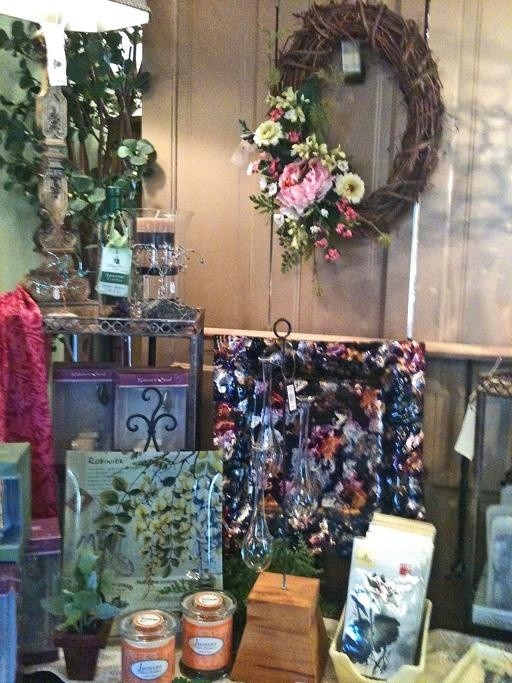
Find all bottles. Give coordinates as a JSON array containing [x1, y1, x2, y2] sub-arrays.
[[122, 587, 237, 683]]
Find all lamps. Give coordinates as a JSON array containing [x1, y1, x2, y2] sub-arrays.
[[0, 0, 153, 306]]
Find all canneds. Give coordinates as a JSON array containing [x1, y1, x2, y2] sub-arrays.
[[119, 610, 178, 683], [178, 589, 237, 681]]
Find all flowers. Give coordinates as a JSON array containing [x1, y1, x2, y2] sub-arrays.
[[231, 26, 397, 275]]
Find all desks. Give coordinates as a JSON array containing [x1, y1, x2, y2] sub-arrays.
[[9, 612, 511, 681], [36, 300, 209, 453]]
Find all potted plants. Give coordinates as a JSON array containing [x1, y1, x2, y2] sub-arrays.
[[40, 549, 129, 682]]
